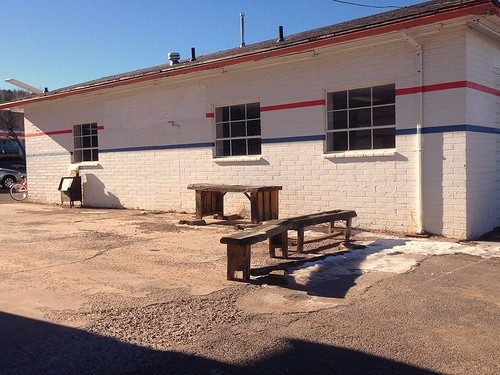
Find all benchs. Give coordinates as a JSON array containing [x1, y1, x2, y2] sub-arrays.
[[283, 209, 359, 253], [220, 219, 290, 283]]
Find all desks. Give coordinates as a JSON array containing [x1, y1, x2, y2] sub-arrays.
[[187, 182, 283, 224]]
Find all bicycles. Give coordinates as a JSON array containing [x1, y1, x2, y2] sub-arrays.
[[10, 176, 28, 201]]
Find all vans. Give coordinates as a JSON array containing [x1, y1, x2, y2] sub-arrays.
[[0, 137, 26, 190]]
